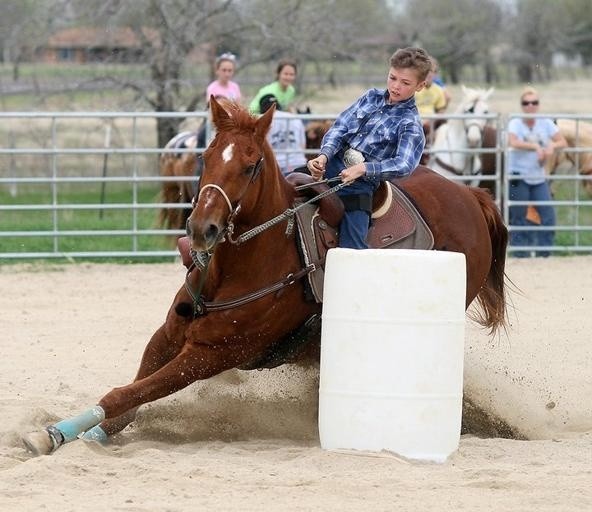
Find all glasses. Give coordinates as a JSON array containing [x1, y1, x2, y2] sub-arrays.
[[522, 100, 538, 106]]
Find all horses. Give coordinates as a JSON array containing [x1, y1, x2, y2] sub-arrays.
[[554, 119, 592, 199], [22, 92, 532, 458], [150, 83, 508, 250]]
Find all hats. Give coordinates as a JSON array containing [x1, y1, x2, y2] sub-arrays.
[[259, 94, 279, 112]]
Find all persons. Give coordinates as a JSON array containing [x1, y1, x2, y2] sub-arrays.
[[206, 50, 241, 117], [505, 86, 568, 257], [308, 46, 434, 249], [249, 60, 298, 112], [413, 56, 451, 114]]
